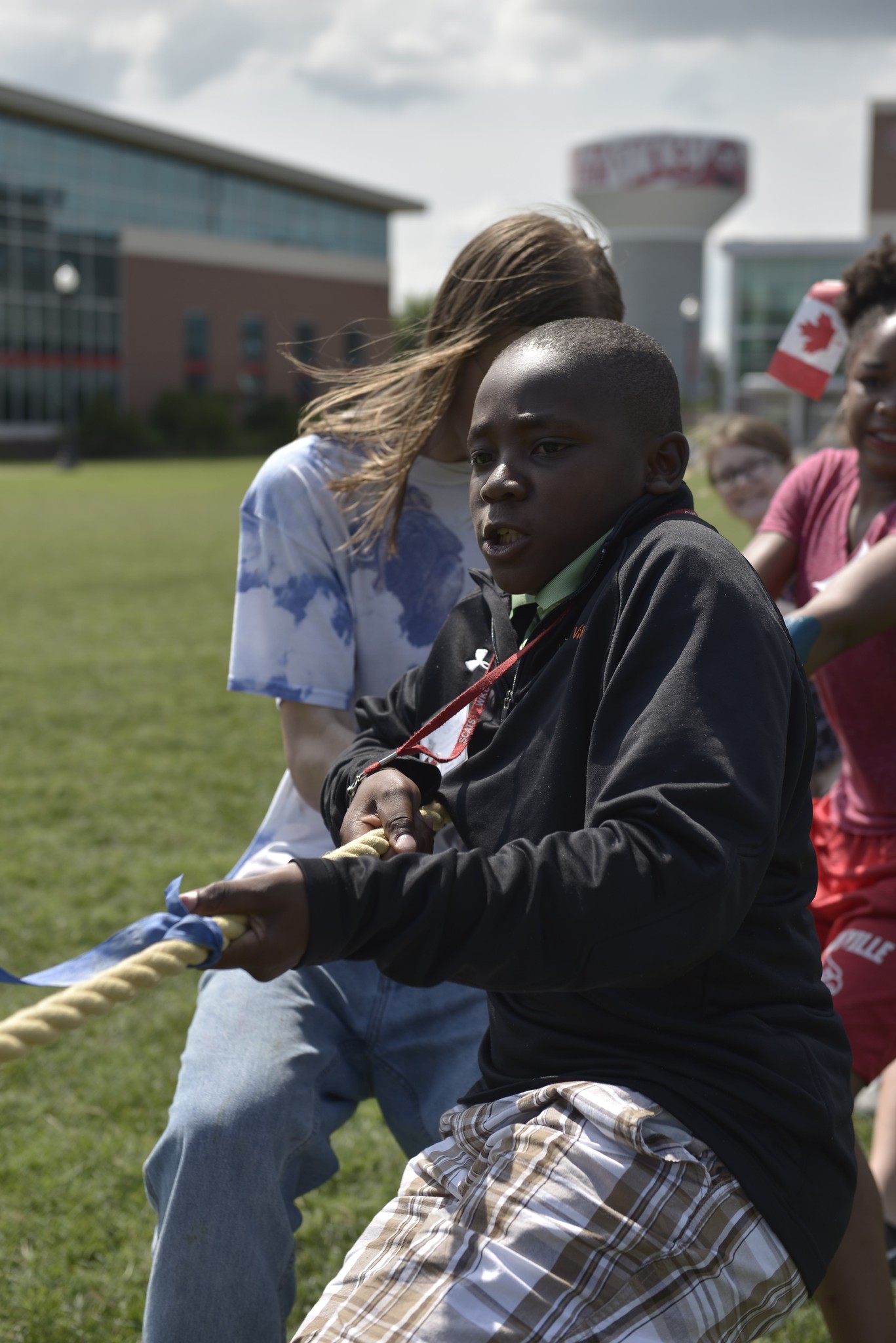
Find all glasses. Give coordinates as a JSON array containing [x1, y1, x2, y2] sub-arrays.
[[709, 455, 778, 492]]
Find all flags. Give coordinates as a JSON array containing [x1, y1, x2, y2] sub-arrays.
[[767, 278, 846, 401]]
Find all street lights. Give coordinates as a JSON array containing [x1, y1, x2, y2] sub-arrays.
[[52, 262, 81, 469], [680, 296, 700, 435]]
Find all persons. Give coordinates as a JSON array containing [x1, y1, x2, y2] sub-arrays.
[[739, 234, 896, 1234], [143, 213, 623, 1343], [178, 320, 853, 1343], [707, 417, 796, 533]]
